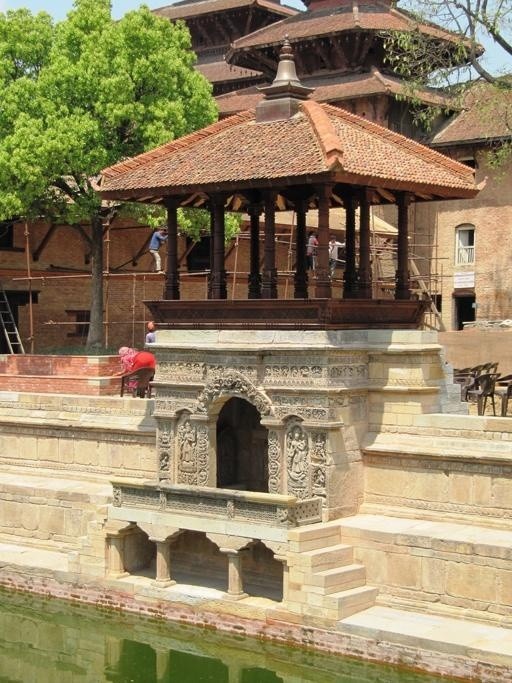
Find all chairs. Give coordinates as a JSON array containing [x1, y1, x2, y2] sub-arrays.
[[454, 362, 512, 416], [121, 367, 155, 399]]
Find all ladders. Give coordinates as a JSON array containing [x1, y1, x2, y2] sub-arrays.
[[0, 289, 25, 354]]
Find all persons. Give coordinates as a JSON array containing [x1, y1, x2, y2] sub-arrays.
[[306, 233, 319, 271], [115, 347, 140, 393], [148, 226, 168, 274], [328, 234, 346, 280], [144, 321, 156, 354]]
